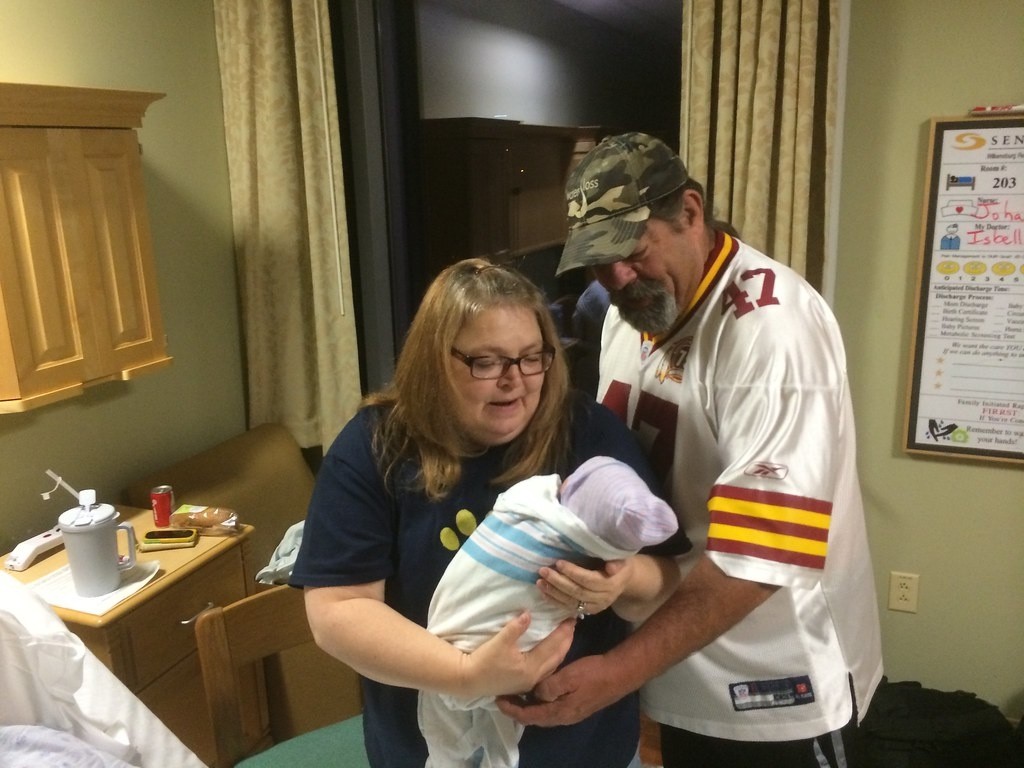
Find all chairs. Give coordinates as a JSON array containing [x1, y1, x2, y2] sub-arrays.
[[193, 584, 371, 768]]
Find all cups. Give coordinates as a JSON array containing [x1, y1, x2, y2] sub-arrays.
[[59, 503, 136, 596]]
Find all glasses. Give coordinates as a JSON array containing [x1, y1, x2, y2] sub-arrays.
[[450, 339, 555, 380]]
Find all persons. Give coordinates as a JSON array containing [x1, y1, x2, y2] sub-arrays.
[[417, 454, 678, 768], [553, 133, 882, 768], [291, 256, 692, 768]]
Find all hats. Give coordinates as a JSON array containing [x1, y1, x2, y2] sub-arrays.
[[553, 132, 689, 274], [559, 455, 679, 551]]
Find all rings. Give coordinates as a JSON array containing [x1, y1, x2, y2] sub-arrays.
[[575, 602, 589, 619]]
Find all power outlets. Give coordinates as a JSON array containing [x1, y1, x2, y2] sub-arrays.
[[886, 570, 920, 615]]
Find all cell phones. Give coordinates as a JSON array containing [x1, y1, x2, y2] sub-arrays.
[[142, 528, 197, 543]]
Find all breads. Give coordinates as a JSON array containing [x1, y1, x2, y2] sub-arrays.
[[169, 504, 241, 535]]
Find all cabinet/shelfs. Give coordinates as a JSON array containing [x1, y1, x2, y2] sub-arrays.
[[0, 81, 174, 414], [0, 505, 274, 768], [422, 116, 615, 267]]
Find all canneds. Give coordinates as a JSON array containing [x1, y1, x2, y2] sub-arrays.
[[150, 484, 174, 527]]
